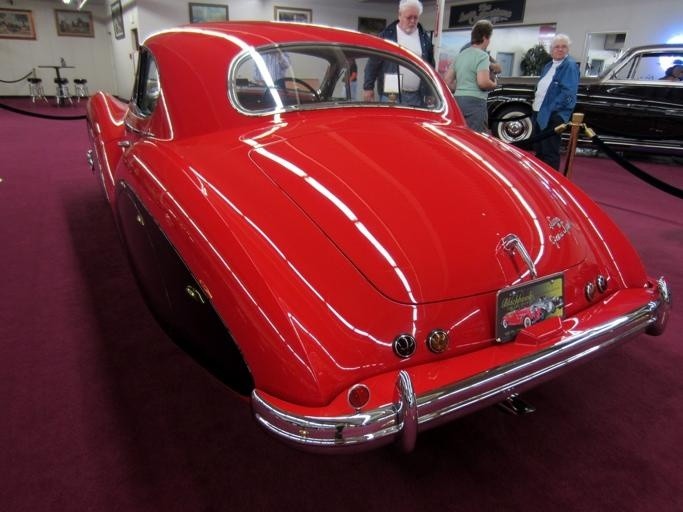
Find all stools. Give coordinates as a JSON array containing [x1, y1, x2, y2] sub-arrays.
[[26, 77, 89, 106]]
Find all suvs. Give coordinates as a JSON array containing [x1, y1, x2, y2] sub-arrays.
[[487, 43, 682, 158]]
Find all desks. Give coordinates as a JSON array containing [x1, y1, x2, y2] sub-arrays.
[[39, 64, 76, 107]]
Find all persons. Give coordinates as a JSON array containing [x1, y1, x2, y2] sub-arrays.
[[658, 65, 683, 82], [531, 33, 579, 172], [342, 60, 358, 101], [250, 52, 291, 88], [444, 20, 502, 134], [363, 0, 436, 108]]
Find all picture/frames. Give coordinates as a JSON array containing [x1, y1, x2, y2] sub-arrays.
[[110, 1, 125, 38], [1, 9, 36, 41], [189, 3, 228, 23], [274, 6, 312, 24], [54, 9, 95, 37]]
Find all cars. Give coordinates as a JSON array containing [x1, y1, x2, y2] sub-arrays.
[[83, 20, 670, 458]]
